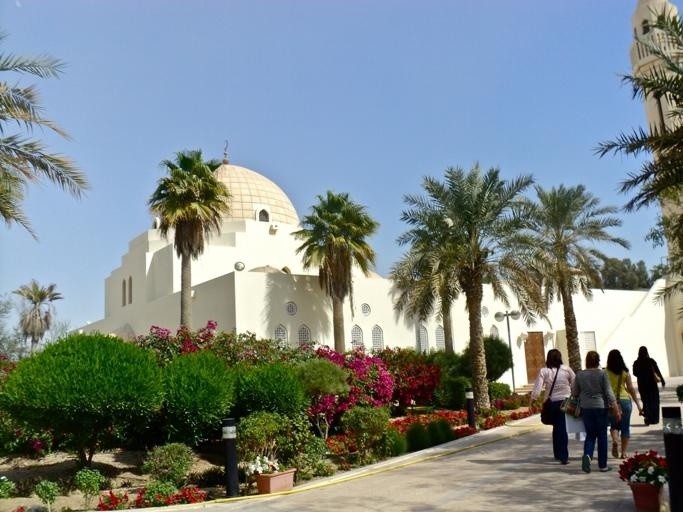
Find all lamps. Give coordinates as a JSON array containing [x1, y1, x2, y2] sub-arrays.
[[519, 332, 528, 343], [546, 331, 554, 338]]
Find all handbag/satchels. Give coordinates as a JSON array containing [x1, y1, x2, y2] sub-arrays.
[[565, 412, 586, 433], [559, 394, 582, 418], [541, 400, 554, 424], [654, 372, 661, 382], [608, 403, 622, 419]]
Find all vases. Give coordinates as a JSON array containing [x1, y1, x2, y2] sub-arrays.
[[629, 483, 661, 512], [256, 467, 297, 494]]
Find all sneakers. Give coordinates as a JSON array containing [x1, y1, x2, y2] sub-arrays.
[[612, 441, 628, 458], [555, 456, 570, 464], [582, 454, 592, 473], [600, 467, 612, 472]]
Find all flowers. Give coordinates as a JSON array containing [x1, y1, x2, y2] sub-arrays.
[[241, 456, 288, 476], [618, 449, 672, 486]]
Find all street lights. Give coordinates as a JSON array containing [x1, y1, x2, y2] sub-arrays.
[[495, 310, 520, 393]]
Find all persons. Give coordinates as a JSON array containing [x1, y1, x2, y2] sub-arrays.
[[632, 346, 665, 425], [573, 351, 622, 473], [530, 348, 576, 465], [603, 349, 642, 458]]
